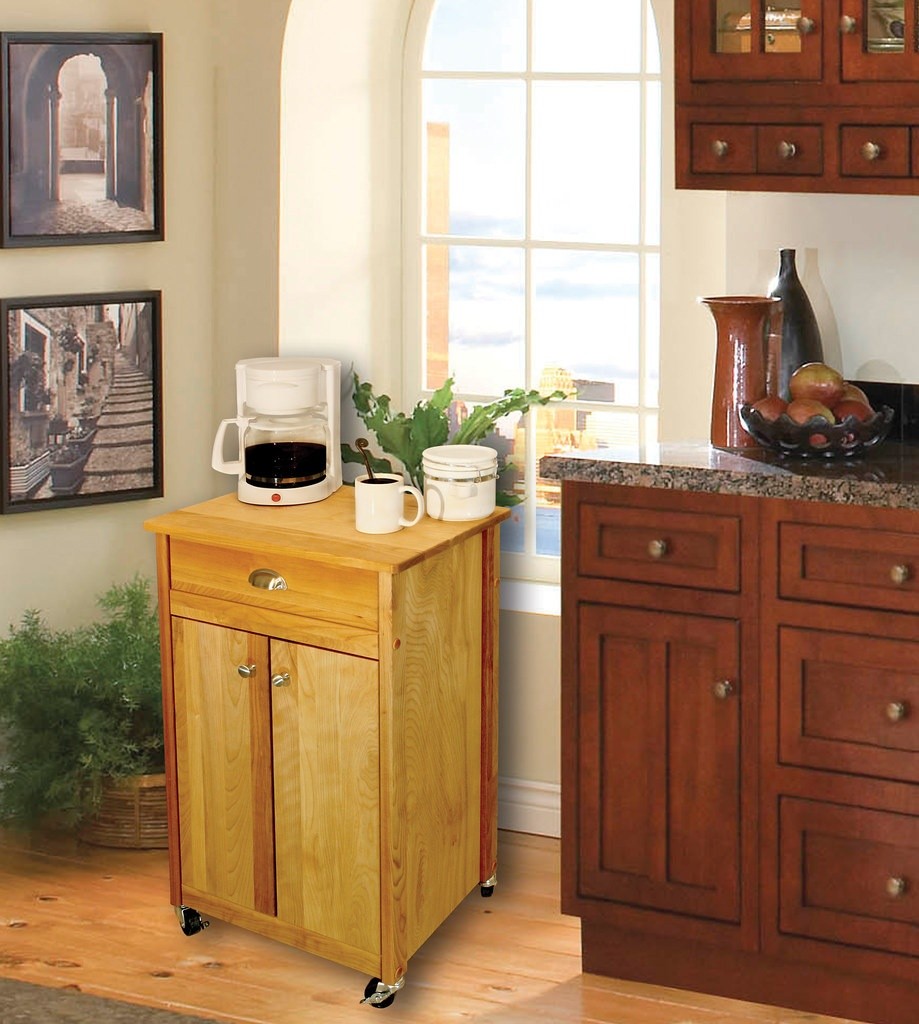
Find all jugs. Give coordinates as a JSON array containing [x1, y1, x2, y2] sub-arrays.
[[211, 409, 328, 486]]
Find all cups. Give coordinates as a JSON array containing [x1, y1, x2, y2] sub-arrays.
[[353, 473, 425, 534]]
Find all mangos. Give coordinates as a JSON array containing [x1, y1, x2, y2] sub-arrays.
[[790, 364, 849, 407], [833, 402, 874, 442], [751, 397, 787, 424], [843, 386, 869, 403], [787, 400, 835, 445]]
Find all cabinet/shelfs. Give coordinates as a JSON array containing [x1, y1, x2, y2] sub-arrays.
[[142, 482, 510, 1012], [561, 488, 918, 1024], [672, 0, 919, 196]]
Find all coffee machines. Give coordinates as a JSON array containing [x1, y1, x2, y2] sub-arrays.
[[236, 356, 344, 508]]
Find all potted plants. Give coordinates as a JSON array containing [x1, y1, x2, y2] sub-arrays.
[[0, 568, 168, 854]]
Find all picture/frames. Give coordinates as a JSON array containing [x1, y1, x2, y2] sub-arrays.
[[0, 31, 165, 249], [0, 288, 164, 514]]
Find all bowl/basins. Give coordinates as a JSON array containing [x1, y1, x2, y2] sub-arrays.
[[422, 444, 498, 522]]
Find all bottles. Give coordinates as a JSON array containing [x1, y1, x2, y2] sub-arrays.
[[765, 247, 822, 403]]
